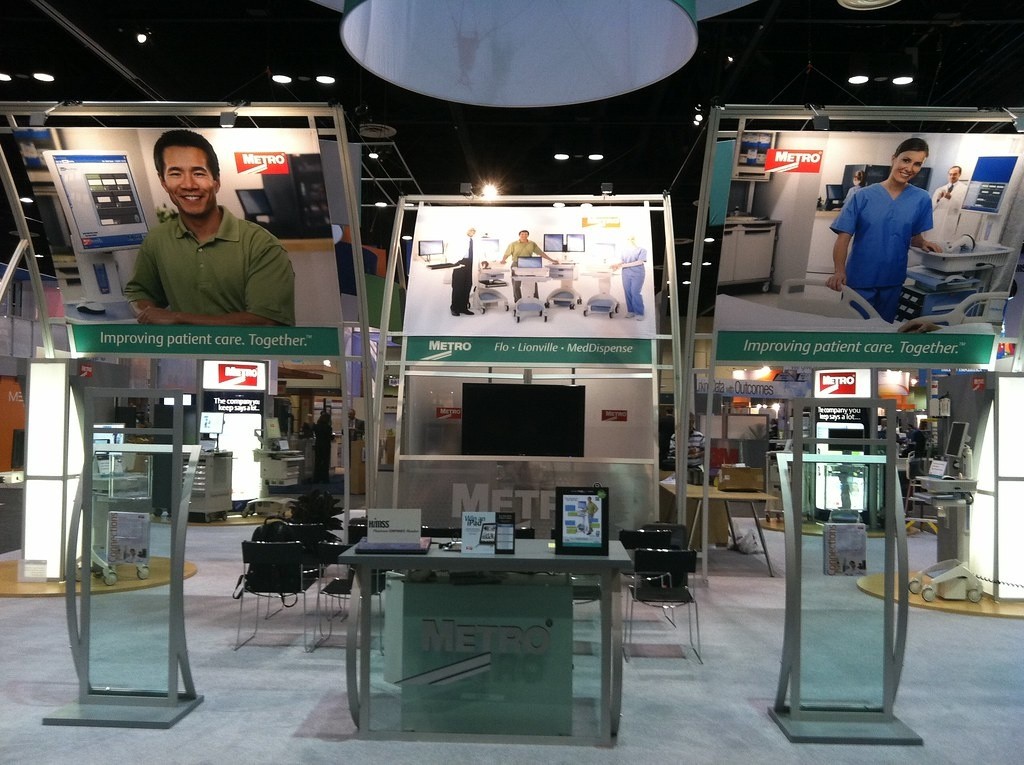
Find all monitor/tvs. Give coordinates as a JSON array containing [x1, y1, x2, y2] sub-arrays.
[[567, 234, 585, 251], [236, 189, 272, 215], [93, 423, 125, 455], [44, 149, 152, 305], [164, 394, 191, 405], [944, 421, 973, 480], [462, 382, 585, 458], [517, 256, 542, 268], [199, 413, 224, 452], [418, 241, 444, 261], [826, 185, 843, 212], [266, 417, 282, 438], [544, 234, 563, 251]]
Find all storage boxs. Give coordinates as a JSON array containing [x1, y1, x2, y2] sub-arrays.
[[717, 467, 764, 490]]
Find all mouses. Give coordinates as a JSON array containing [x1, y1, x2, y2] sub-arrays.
[[74, 301, 106, 315], [942, 475, 956, 480]]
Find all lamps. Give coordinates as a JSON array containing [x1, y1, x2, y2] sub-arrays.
[[29, 100, 64, 125], [600, 183, 612, 194], [459, 183, 474, 195], [806, 103, 829, 132], [1001, 107, 1023, 133], [219, 111, 238, 128]]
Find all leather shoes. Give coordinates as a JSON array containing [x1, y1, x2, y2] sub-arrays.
[[460, 309, 474, 315], [451, 308, 460, 316]]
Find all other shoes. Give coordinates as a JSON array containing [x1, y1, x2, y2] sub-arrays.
[[624, 311, 635, 319], [635, 314, 643, 321]]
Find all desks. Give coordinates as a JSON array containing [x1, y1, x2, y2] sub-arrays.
[[337, 537, 631, 740], [658, 481, 779, 577]]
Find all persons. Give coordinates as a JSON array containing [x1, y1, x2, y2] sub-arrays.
[[906, 423, 916, 438], [611, 235, 648, 320], [123, 129, 296, 326], [826, 138, 942, 324], [301, 413, 316, 438], [843, 169, 867, 204], [911, 419, 928, 458], [347, 408, 365, 441], [581, 497, 598, 535], [923, 166, 967, 243], [450, 226, 476, 316], [314, 413, 335, 483], [878, 418, 887, 431], [667, 412, 705, 468], [658, 408, 675, 457], [500, 231, 558, 303]]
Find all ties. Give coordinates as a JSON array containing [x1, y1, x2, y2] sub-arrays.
[[349, 420, 354, 428], [947, 184, 954, 193]]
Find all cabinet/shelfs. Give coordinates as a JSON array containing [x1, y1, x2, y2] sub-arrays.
[[718, 221, 776, 287]]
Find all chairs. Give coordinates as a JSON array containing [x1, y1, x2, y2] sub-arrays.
[[617, 521, 700, 662], [232, 515, 392, 656]]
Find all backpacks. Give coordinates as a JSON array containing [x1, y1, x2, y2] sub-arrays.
[[245, 515, 299, 593]]
